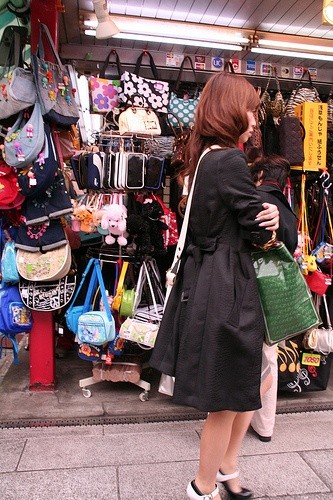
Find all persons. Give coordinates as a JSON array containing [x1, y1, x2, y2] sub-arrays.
[[249, 157, 299, 442], [150, 72, 279, 500]]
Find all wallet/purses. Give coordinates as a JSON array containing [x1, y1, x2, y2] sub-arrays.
[[70, 151, 167, 191]]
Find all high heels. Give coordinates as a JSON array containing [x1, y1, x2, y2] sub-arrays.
[[187, 479, 222, 500], [215, 468, 252, 500]]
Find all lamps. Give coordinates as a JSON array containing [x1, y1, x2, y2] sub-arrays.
[[322, 0, 333, 27], [92, 0, 120, 40]]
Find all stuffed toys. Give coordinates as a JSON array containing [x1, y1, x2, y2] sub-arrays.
[[294, 230, 317, 276], [73, 200, 169, 258]]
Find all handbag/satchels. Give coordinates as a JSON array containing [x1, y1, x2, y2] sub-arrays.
[[277, 334, 330, 393], [89, 49, 204, 161], [65, 256, 167, 365], [250, 241, 323, 347], [0, 19, 81, 312], [302, 328, 333, 355], [245, 69, 333, 171], [304, 270, 332, 296]]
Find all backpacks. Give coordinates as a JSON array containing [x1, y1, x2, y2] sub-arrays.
[[0, 279, 33, 366]]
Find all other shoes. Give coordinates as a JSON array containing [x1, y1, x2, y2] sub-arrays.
[[247, 423, 271, 442]]
